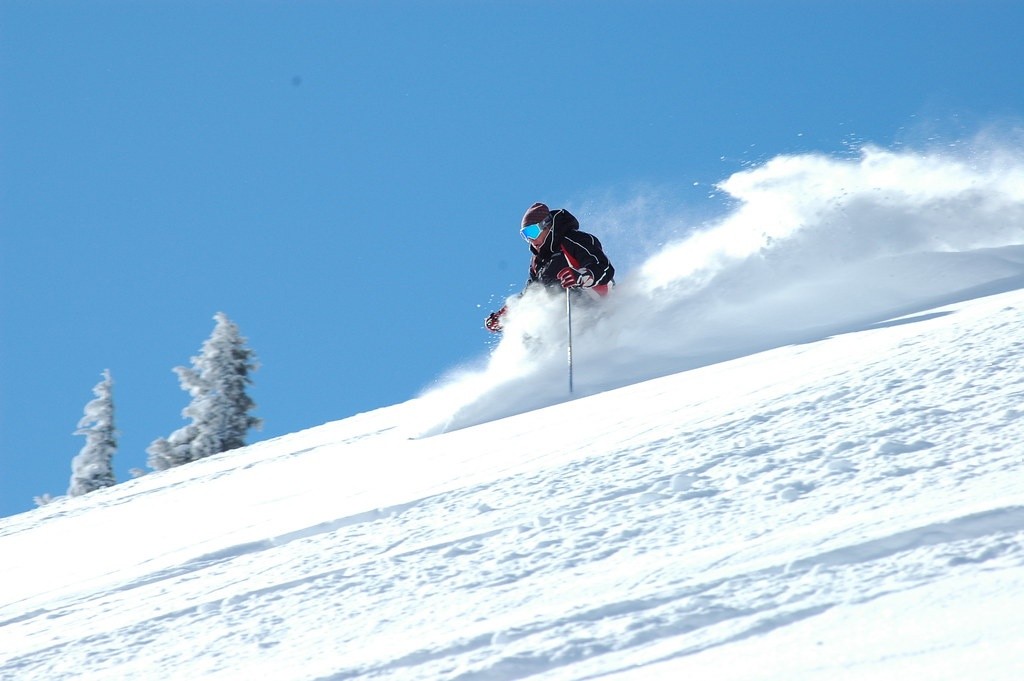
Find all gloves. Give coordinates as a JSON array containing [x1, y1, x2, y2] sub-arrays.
[[483, 306, 506, 332], [557, 266, 593, 288]]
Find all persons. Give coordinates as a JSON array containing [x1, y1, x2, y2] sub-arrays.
[[485, 203, 616, 332]]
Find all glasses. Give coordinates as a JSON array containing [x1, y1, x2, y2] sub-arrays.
[[519, 215, 551, 245]]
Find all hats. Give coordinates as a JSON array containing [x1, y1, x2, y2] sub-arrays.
[[520, 202, 553, 226]]
